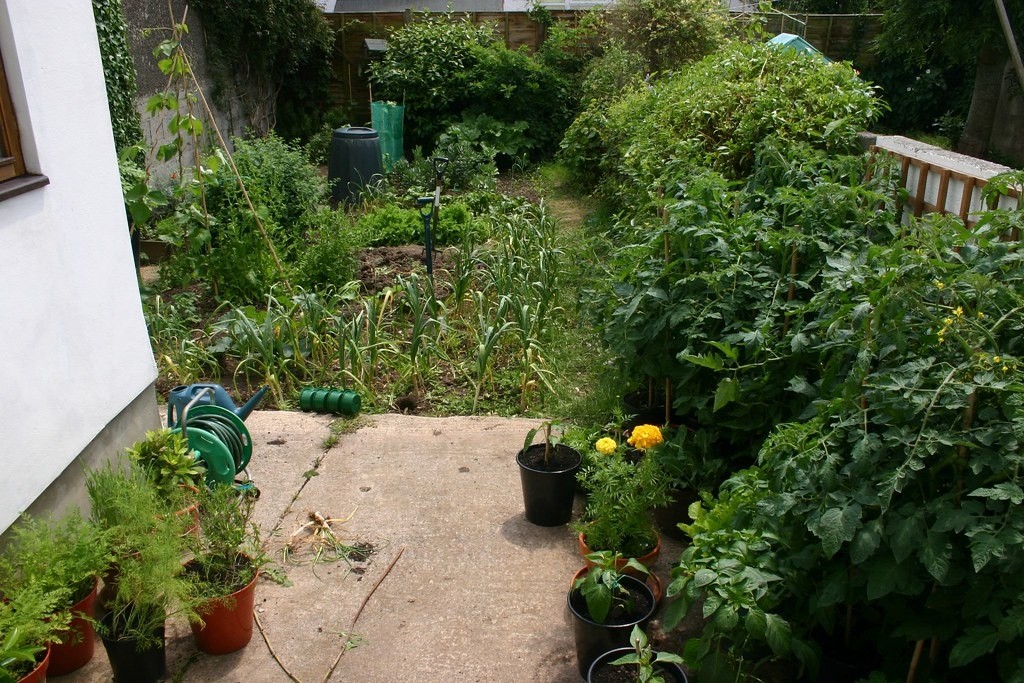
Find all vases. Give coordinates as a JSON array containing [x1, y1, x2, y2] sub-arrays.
[[578, 520, 661, 584]]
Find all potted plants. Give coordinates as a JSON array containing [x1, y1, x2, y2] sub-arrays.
[[566, 550, 656, 681], [0, 427, 296, 683], [587, 624, 688, 683], [516, 419, 584, 527]]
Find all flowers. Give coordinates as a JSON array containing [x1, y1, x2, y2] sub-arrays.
[[574, 394, 688, 556]]
